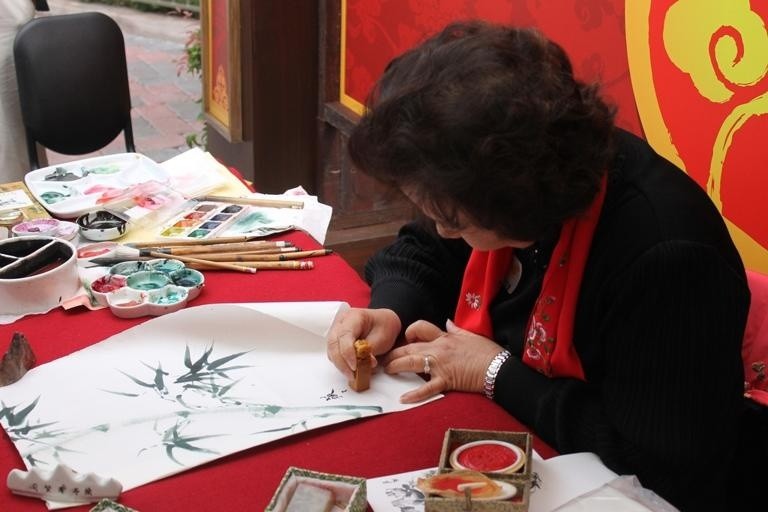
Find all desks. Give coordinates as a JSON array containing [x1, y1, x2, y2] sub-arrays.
[[1, 161, 683, 511]]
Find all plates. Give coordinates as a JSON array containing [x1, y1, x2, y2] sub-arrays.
[[10, 218, 77, 243]]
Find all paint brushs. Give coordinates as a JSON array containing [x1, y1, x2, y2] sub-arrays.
[[88, 236, 333, 274], [189, 195, 305, 209]]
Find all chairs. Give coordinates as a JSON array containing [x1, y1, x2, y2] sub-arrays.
[[13, 13, 135, 171]]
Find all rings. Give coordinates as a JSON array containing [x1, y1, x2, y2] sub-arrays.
[[420, 352, 440, 374]]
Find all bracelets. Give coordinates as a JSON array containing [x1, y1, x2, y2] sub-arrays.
[[484, 347, 511, 402]]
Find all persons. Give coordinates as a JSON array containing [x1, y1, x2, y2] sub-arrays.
[[328, 18, 750, 512]]
